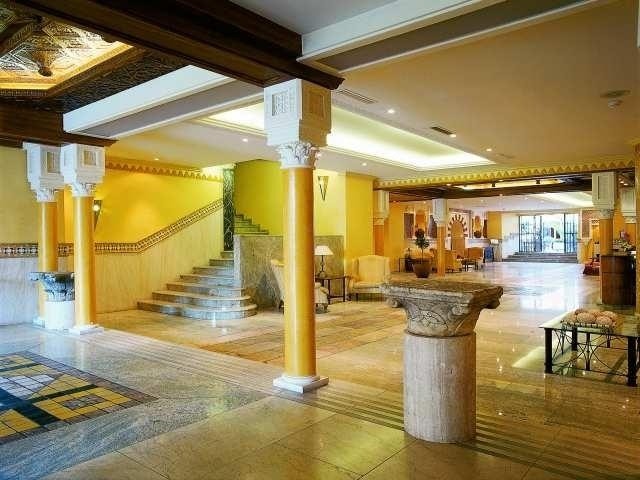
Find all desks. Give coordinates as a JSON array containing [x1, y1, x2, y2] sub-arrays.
[[600, 251, 634, 306], [538, 305, 640, 386]]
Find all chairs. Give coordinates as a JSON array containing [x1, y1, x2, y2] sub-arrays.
[[349, 254, 392, 301], [430, 247, 463, 273], [406, 249, 430, 272], [271, 258, 329, 313], [463, 247, 484, 270]]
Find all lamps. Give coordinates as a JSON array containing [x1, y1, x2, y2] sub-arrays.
[[314, 245, 334, 278]]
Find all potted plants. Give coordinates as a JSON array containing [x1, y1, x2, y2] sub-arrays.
[[412, 228, 432, 278]]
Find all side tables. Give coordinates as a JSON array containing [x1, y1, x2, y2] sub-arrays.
[[315, 274, 350, 304]]
